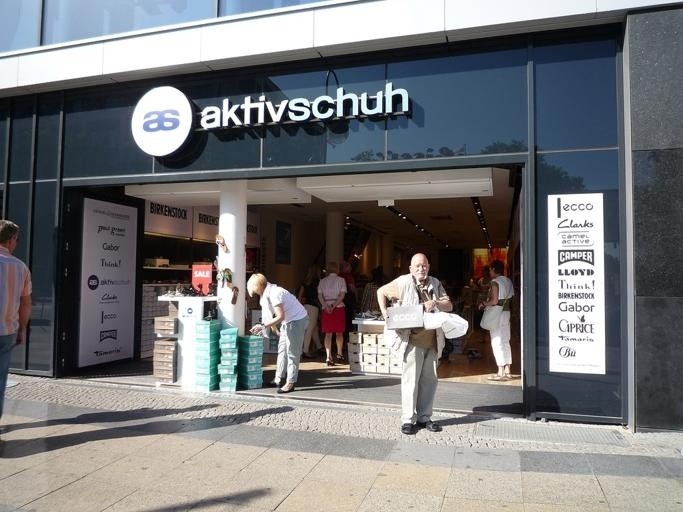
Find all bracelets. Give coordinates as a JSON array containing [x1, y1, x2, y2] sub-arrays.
[[480, 302, 484, 306], [261, 322, 266, 330], [432, 300, 436, 309]]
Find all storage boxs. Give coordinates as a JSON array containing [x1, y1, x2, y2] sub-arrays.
[[196, 320, 221, 391], [347, 331, 403, 374], [451, 338, 464, 354], [240, 336, 263, 389], [142, 287, 178, 317], [141, 319, 155, 352], [217, 328, 239, 390], [154, 316, 177, 335], [152, 339, 178, 383]]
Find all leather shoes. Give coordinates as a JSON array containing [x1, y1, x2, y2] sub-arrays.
[[304, 352, 317, 359], [416, 421, 442, 432], [265, 382, 281, 387], [401, 423, 416, 434], [277, 385, 294, 393]]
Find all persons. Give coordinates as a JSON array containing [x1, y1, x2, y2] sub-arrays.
[[245, 272, 311, 394], [0, 218, 33, 420], [295, 259, 404, 368], [376, 252, 456, 434], [478, 258, 515, 376], [475, 263, 492, 343], [459, 277, 478, 318]]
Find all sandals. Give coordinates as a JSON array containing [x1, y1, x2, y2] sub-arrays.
[[504, 372, 512, 380], [487, 374, 505, 381], [325, 357, 334, 366], [336, 353, 346, 362]]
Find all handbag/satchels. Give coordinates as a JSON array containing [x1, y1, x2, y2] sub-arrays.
[[479, 302, 503, 332]]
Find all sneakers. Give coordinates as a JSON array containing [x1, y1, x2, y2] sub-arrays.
[[355, 310, 379, 320]]
